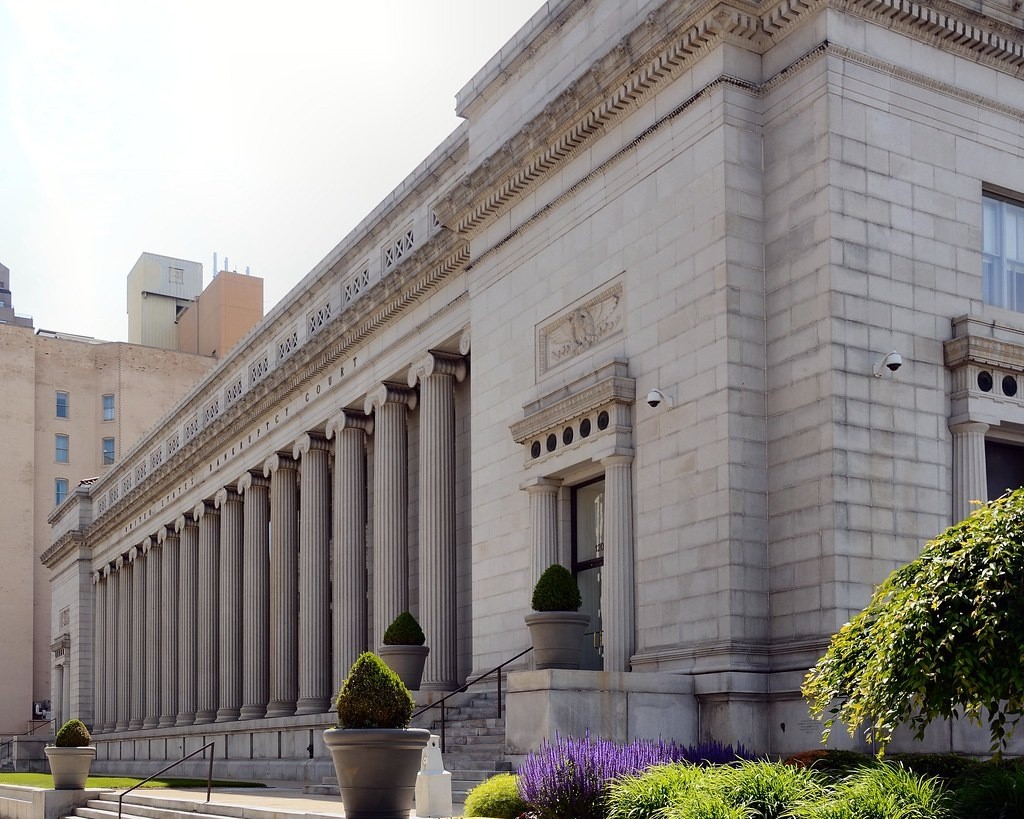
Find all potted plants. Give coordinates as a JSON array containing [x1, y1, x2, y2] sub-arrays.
[[380, 611, 431, 691], [522, 564, 593, 670], [322, 651, 431, 819], [43, 719, 96, 790]]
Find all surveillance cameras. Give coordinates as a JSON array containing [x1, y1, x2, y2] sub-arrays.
[[886, 353, 902, 371], [647, 392, 661, 407]]
